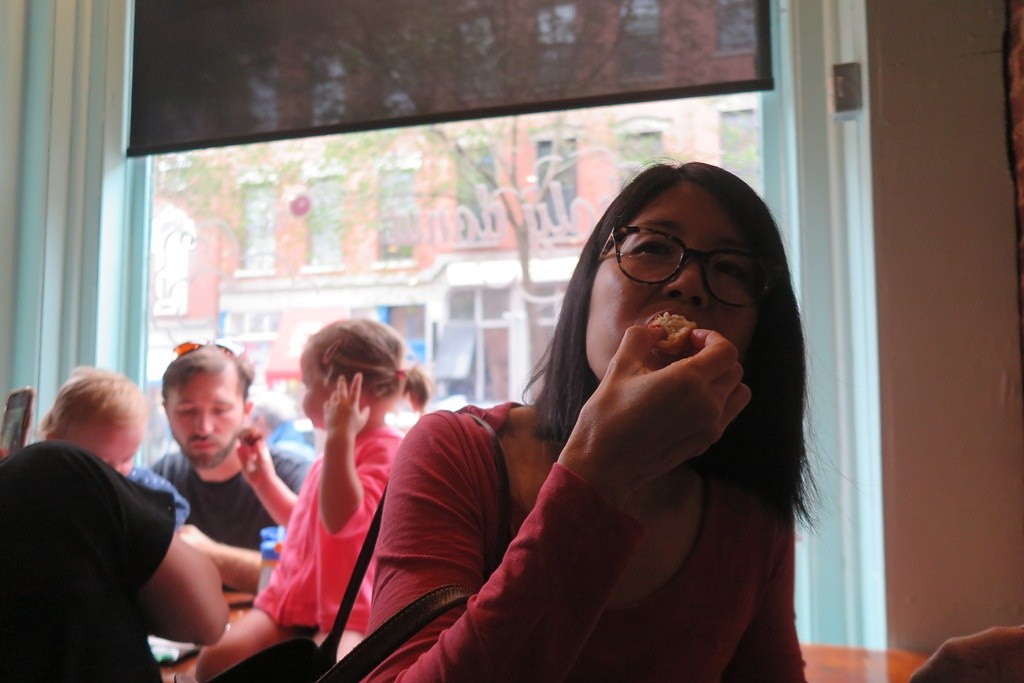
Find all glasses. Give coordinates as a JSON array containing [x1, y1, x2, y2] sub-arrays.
[[597, 225, 773, 307], [170, 342, 235, 363]]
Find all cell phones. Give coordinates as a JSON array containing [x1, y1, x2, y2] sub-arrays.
[[0, 388, 33, 462]]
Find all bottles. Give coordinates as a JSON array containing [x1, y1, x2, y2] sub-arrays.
[[256, 525, 287, 607]]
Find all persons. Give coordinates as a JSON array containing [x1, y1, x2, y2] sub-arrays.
[[197, 317, 428, 682], [361, 159, 806, 683], [38, 369, 190, 529], [0, 440, 230, 683], [152, 343, 316, 596]]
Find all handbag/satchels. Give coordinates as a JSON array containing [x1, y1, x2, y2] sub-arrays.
[[196, 412, 510, 683]]
